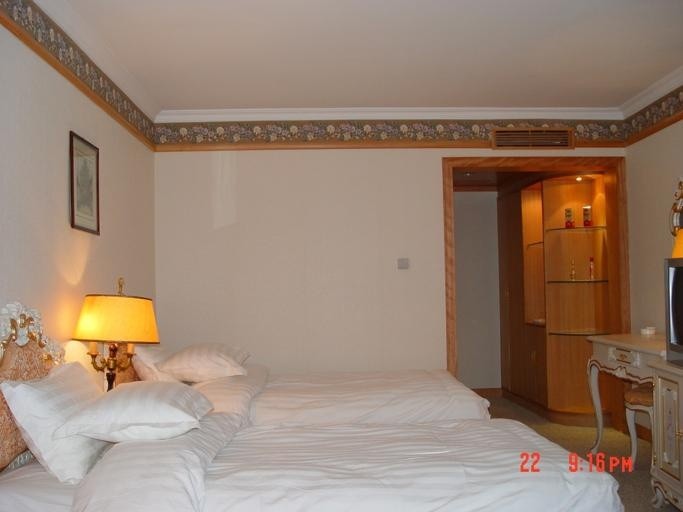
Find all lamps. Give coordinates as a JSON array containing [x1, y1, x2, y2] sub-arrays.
[[72, 278, 160, 390]]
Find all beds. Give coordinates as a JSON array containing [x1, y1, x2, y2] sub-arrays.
[[108, 324, 491, 422], [0, 300, 627, 512]]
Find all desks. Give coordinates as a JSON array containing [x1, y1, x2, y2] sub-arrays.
[[585, 332, 667, 456]]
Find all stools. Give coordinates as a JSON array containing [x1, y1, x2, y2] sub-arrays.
[[624, 384, 654, 465]]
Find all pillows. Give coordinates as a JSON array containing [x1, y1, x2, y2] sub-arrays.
[[0, 342, 248, 482]]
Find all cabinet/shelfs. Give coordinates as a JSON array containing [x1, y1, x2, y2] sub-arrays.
[[497, 171, 619, 428], [646, 358, 683, 511]]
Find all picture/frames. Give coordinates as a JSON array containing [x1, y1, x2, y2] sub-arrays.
[[69, 130, 99, 234]]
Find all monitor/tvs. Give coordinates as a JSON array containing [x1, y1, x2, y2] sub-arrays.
[[663, 258, 683, 366]]
